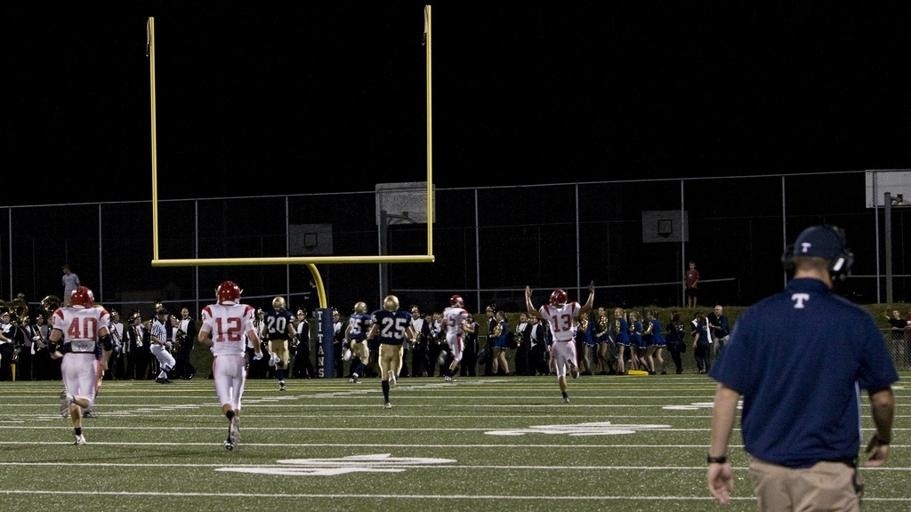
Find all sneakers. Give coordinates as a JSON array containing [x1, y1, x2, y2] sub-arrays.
[[561, 397, 570, 404], [387, 369, 396, 388], [445, 375, 457, 382], [279, 380, 285, 392], [224, 415, 240, 452], [155, 377, 174, 385], [567, 359, 578, 379], [382, 401, 392, 410], [59, 390, 70, 419], [73, 433, 88, 446], [160, 366, 175, 377], [348, 375, 362, 383]]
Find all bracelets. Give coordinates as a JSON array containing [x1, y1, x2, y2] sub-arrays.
[[707, 456, 726, 463], [875, 433, 890, 444]]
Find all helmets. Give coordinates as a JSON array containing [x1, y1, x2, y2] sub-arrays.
[[70, 285, 94, 308], [382, 295, 400, 312], [549, 288, 567, 306], [215, 280, 240, 304], [272, 296, 286, 311], [353, 301, 368, 315], [450, 294, 465, 308]]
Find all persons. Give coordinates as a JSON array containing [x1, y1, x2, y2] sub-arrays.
[[367, 295, 413, 410], [526, 282, 594, 403], [879, 308, 911, 369], [48, 286, 114, 446], [198, 281, 263, 451], [905, 310, 911, 369], [575, 305, 730, 375], [706, 225, 900, 512], [685, 262, 699, 309], [398, 295, 554, 382], [0, 293, 64, 382], [61, 264, 81, 308], [244, 297, 378, 392], [103, 303, 197, 383]]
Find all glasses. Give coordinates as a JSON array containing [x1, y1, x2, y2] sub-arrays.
[[296, 312, 305, 316]]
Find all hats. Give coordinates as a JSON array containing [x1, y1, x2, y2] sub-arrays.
[[795, 226, 845, 266], [158, 309, 171, 315]]
